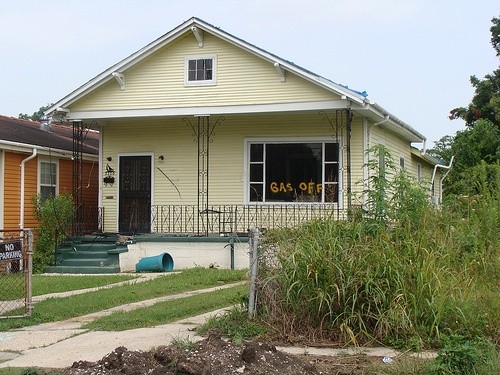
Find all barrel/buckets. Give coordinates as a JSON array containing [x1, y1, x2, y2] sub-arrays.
[[136, 251, 174, 272]]
